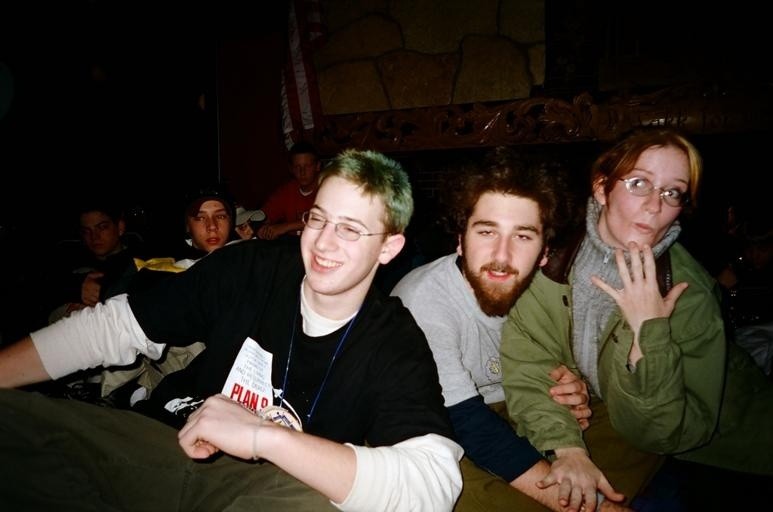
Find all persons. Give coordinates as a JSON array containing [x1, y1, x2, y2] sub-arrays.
[[0, 148, 464, 512], [728, 205, 771, 325], [40, 144, 326, 310], [390, 167, 625, 511], [499, 131, 727, 510]]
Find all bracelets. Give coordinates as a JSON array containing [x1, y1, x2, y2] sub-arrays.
[[249, 416, 266, 461]]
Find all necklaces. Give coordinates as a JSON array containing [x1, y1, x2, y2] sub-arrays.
[[279, 308, 357, 419]]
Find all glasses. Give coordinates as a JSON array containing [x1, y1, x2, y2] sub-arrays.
[[303, 212, 389, 241], [619, 176, 687, 207]]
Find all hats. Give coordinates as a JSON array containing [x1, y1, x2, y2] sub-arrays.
[[184, 186, 235, 217]]
